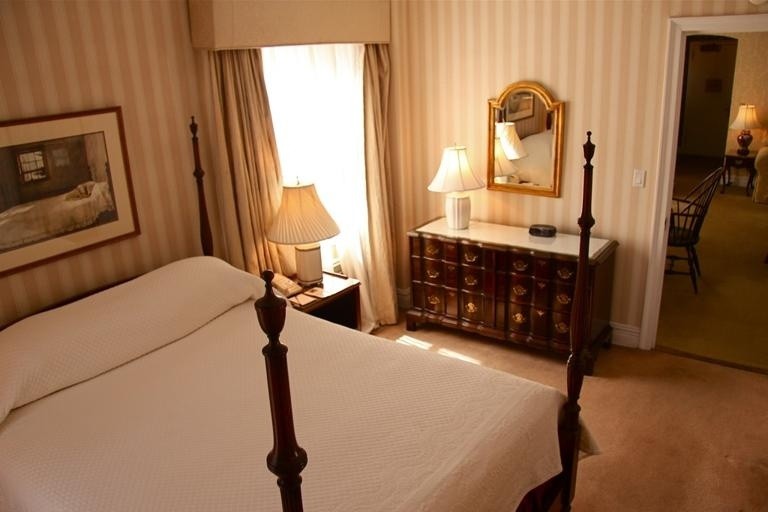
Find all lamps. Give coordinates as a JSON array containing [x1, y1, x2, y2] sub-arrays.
[[428, 145, 486, 230], [729, 105, 762, 155], [494, 138, 519, 176], [265, 176, 340, 286], [494, 122, 528, 184]]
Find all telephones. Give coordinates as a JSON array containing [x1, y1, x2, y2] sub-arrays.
[[270, 272, 303, 297]]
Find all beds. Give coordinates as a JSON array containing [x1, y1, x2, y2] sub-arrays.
[[0, 116, 595, 512], [511, 111, 556, 187]]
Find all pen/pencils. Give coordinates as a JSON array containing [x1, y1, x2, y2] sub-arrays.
[[295, 296, 301, 306]]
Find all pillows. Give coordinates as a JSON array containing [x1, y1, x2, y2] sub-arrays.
[[0, 256, 294, 423]]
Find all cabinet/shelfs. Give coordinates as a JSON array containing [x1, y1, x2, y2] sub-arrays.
[[407, 215, 619, 376]]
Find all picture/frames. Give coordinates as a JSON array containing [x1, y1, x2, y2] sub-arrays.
[[504, 92, 535, 122], [0, 107, 140, 278]]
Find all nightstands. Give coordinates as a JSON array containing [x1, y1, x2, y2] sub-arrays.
[[288, 270, 361, 330]]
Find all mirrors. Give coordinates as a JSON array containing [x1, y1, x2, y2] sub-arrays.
[[488, 81, 565, 198]]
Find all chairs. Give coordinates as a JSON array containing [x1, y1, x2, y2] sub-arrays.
[[752, 138, 768, 206], [665, 167, 725, 294]]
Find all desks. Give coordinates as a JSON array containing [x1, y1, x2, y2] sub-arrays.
[[720, 149, 758, 196]]
